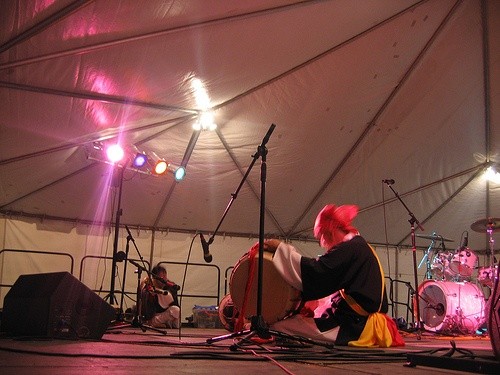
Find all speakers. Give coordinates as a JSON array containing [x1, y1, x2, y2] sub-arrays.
[[3, 271, 116, 342], [484, 279, 500, 356]]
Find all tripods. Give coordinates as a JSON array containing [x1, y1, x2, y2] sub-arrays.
[[206, 124, 333, 350], [387, 184, 458, 341], [107, 260, 175, 334]]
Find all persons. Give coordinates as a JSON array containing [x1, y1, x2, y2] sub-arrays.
[[139, 266, 180, 329], [262, 203, 388, 346]]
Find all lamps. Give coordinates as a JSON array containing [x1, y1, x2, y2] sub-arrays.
[[124, 144, 148, 168], [147, 151, 167, 175], [167, 162, 185, 181]]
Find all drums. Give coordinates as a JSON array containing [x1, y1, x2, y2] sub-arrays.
[[218, 294, 251, 331], [228, 250, 304, 323], [449, 245, 479, 276], [431, 249, 454, 279], [414, 278, 485, 334], [477, 264, 498, 286]]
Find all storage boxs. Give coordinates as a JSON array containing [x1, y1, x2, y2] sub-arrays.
[[192, 309, 218, 328]]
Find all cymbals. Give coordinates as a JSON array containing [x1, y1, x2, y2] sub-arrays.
[[471, 217, 500, 233], [415, 234, 455, 242]]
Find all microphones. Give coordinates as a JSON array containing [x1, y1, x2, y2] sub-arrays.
[[150, 289, 168, 295], [464, 233, 469, 247], [199, 233, 212, 263], [384, 179, 394, 185], [486, 250, 497, 263], [173, 285, 180, 289], [440, 237, 446, 251]]
